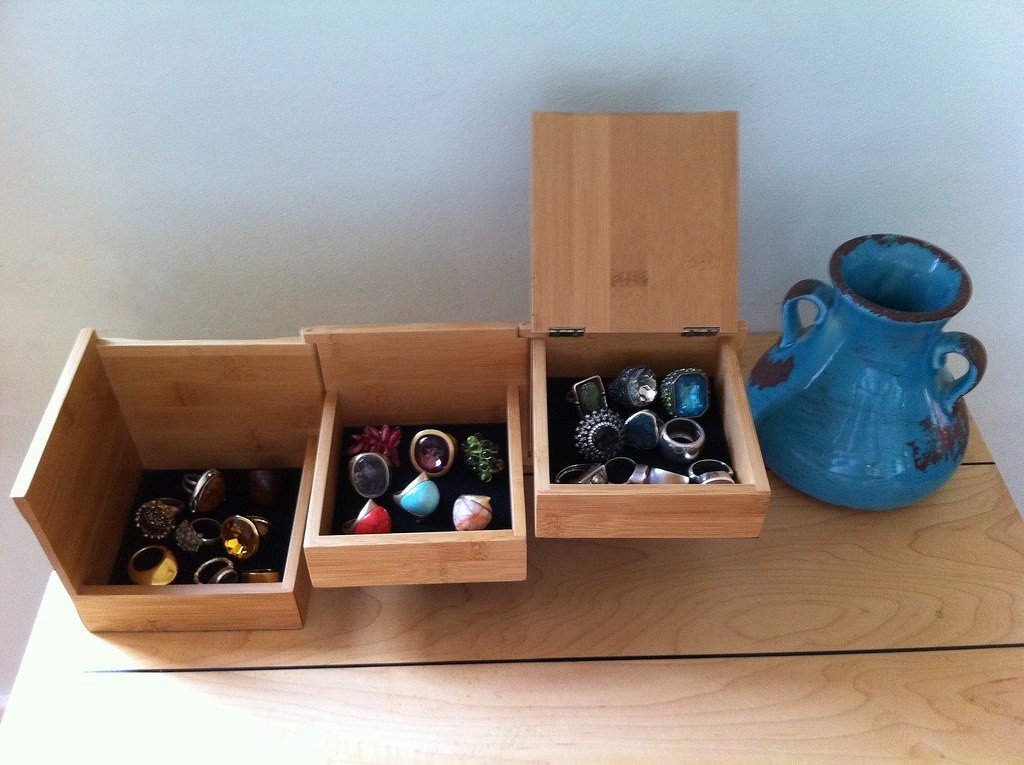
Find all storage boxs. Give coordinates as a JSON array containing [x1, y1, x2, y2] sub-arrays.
[[8, 108, 773, 632]]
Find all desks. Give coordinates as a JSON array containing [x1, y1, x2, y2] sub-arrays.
[[0, 332, 1024, 763]]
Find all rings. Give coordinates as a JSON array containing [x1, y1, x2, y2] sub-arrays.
[[341, 499, 391, 534], [453, 494, 493, 530], [129, 470, 280, 586], [462, 433, 504, 482], [557, 364, 736, 485], [409, 429, 457, 477], [347, 452, 395, 499], [348, 424, 402, 466], [393, 471, 439, 516]]
